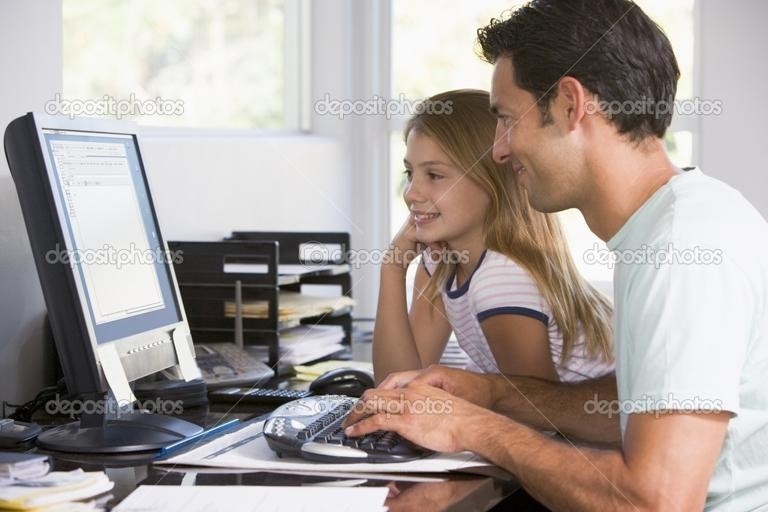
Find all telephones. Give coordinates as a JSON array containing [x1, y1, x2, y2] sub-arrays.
[[160, 280, 274, 390]]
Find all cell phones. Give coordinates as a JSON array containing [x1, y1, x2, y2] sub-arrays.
[[211, 383, 310, 403]]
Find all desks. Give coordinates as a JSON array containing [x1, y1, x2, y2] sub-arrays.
[[0, 372, 521, 510]]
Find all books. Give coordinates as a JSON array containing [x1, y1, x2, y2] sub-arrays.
[[0, 448, 119, 512], [221, 257, 355, 369]]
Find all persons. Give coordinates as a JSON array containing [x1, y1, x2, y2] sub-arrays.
[[337, 1, 767, 511], [366, 85, 619, 391], [382, 467, 493, 511]]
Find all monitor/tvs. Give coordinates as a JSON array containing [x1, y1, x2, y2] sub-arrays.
[[0, 109, 210, 457]]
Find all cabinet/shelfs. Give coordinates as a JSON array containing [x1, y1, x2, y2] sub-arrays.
[[167, 231, 355, 372]]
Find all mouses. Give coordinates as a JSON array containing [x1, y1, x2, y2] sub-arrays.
[[312, 366, 375, 397]]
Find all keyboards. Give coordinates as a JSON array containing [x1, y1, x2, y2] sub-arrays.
[[261, 391, 433, 464]]
